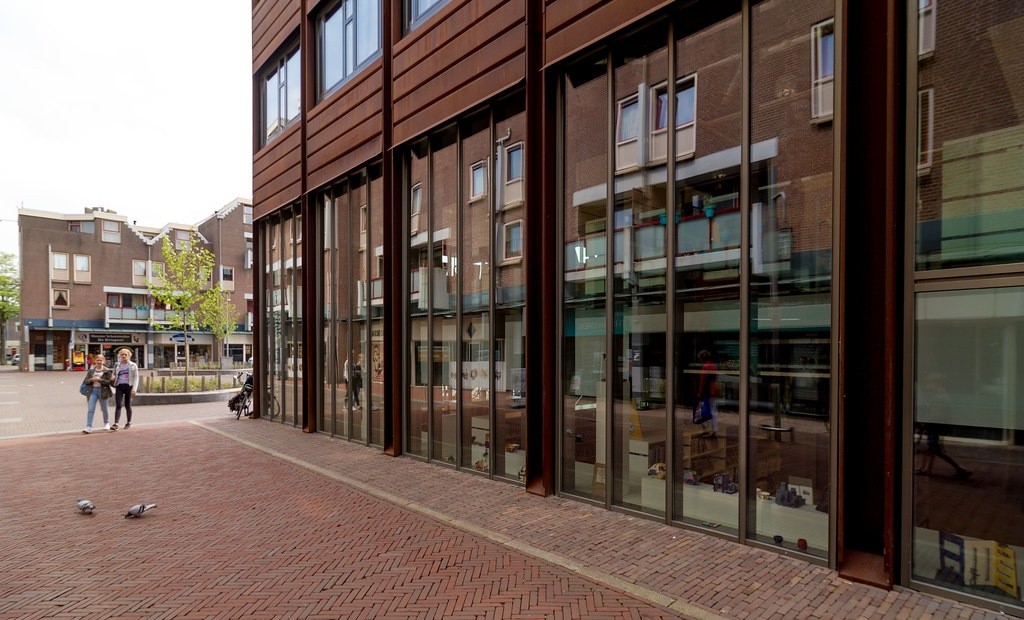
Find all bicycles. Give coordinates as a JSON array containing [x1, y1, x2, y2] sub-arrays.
[[234, 371, 253, 420], [267, 370, 280, 417]]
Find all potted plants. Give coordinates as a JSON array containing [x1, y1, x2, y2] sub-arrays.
[[658, 204, 683, 225], [703, 193, 716, 218]]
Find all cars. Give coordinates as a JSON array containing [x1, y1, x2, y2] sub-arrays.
[[11, 353, 20, 365], [6, 354, 11, 360]]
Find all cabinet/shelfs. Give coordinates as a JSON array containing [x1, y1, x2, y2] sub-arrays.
[[630, 417, 782, 494], [441, 404, 596, 472]]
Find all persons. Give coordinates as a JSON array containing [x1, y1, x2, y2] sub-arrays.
[[110, 349, 139, 430], [343, 351, 378, 412], [81, 355, 113, 435], [698, 350, 720, 442]]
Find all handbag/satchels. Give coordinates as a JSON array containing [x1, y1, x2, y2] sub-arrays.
[[80, 369, 92, 395], [111, 362, 118, 387], [693, 397, 712, 424]]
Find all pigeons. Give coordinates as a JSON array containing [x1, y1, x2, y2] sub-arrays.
[[76, 497, 96, 513], [125, 503, 158, 519]]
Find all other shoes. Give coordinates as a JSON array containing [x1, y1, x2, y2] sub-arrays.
[[110, 424, 119, 431], [104, 423, 110, 430], [83, 426, 92, 434], [124, 423, 132, 429]]
